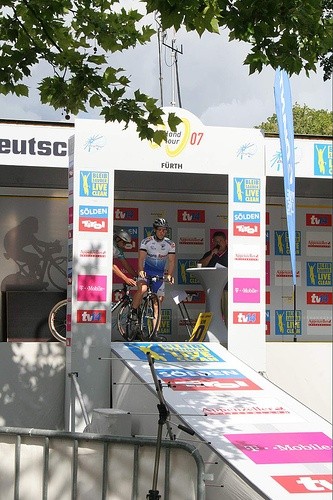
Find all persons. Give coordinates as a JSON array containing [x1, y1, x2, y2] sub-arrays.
[[113, 231, 138, 287], [126, 217, 176, 341], [199, 231, 228, 328]]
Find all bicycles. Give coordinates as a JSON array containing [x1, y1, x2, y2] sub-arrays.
[[126, 272, 173, 341], [48, 277, 137, 342]]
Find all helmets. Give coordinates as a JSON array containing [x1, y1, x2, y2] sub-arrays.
[[153, 218, 168, 230], [116, 229, 132, 243]]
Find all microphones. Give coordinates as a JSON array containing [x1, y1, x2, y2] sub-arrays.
[[213, 245, 220, 256]]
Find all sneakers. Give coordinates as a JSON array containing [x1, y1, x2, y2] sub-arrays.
[[152, 336, 163, 342], [131, 307, 138, 321]]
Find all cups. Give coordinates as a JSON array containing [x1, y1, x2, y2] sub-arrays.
[[197, 263, 202, 268]]
